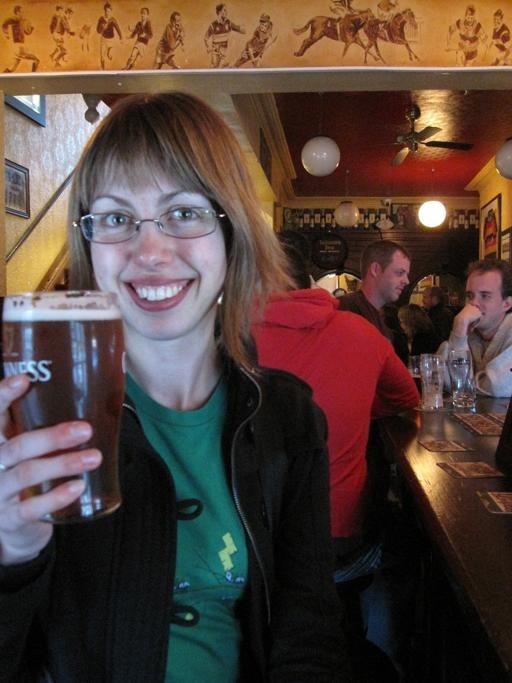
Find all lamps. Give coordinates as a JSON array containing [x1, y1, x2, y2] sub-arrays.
[[300, 96, 341, 177], [417, 170, 448, 229], [333, 172, 359, 227], [492, 96, 512, 179]]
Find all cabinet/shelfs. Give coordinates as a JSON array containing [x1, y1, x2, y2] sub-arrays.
[[288, 228, 479, 298]]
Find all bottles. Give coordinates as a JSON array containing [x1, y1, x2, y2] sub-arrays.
[[483, 208, 497, 262]]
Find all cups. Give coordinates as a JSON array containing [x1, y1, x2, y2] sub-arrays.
[[408, 350, 478, 413], [1, 288, 125, 525]]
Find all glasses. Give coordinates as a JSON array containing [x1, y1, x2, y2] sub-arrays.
[[73, 205, 228, 244]]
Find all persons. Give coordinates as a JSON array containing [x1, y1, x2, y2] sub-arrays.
[[420, 286, 455, 326], [334, 239, 412, 364], [244, 242, 420, 664], [0, 88, 353, 681], [437, 258, 512, 396], [397, 303, 434, 354], [496, 396, 512, 473]]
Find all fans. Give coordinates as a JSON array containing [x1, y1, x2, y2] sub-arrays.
[[370, 105, 474, 166]]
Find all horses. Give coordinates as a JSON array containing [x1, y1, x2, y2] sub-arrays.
[[293, 8, 381, 62], [363, 8, 421, 64]]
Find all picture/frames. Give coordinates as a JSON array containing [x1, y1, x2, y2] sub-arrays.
[[391, 203, 441, 229], [480, 194, 500, 262], [498, 227, 512, 266], [5, 93, 45, 126], [3, 159, 30, 218]]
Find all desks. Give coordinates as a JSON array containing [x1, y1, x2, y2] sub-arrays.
[[376, 386, 512, 683]]
